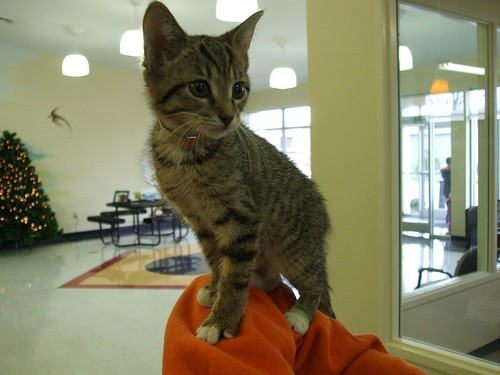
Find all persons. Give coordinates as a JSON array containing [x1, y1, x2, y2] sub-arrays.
[[439, 157, 451, 235], [162, 272, 428, 375]]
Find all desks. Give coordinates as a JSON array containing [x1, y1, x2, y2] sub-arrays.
[[106, 199, 168, 243]]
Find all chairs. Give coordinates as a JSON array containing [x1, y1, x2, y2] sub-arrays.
[[414, 247, 477, 290]]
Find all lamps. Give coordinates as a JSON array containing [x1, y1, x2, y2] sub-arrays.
[[398, 9, 413, 71], [61, 26, 89, 77], [119, 0, 145, 56], [269, 37, 297, 89]]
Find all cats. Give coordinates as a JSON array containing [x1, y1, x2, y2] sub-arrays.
[[141, 1, 337, 345]]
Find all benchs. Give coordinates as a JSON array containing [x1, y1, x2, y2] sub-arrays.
[[87, 209, 189, 247]]
